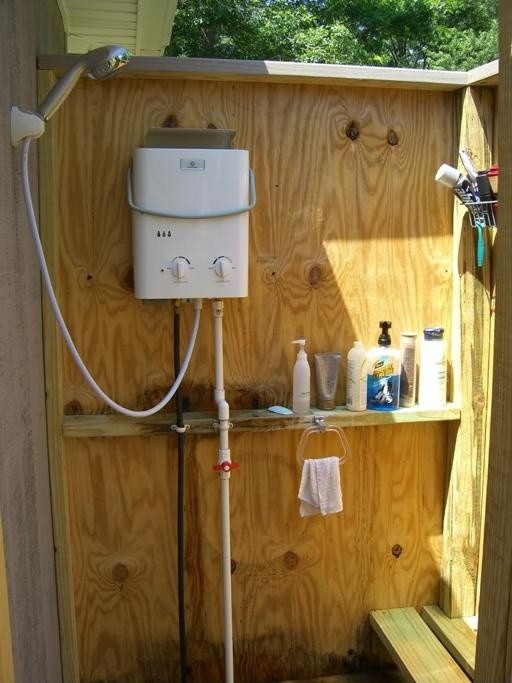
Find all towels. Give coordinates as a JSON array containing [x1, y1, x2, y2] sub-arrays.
[[296, 456, 343, 517]]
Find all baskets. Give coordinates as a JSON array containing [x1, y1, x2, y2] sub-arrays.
[[461, 201, 498, 229]]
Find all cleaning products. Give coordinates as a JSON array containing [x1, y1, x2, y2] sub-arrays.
[[345, 338, 367, 412]]
